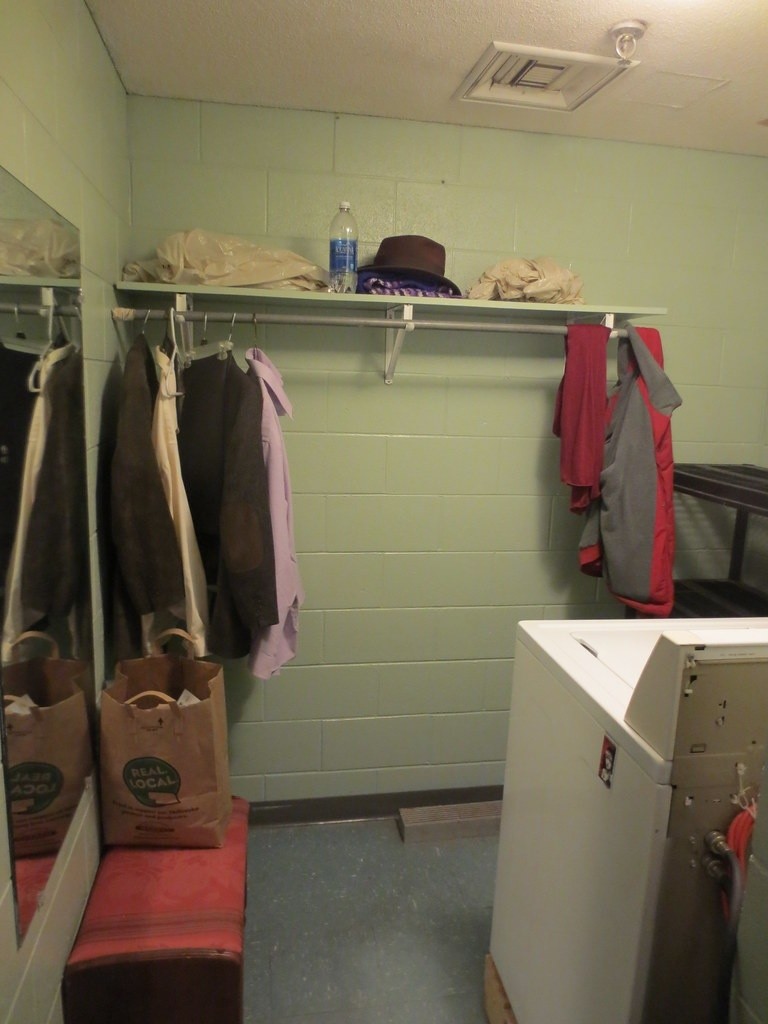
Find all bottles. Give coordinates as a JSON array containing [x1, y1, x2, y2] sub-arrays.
[[328, 200, 359, 293]]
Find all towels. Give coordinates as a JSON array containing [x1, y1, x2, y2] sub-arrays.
[[553, 325, 611, 515]]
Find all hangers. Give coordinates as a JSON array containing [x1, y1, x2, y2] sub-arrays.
[[0, 302, 72, 392], [126, 306, 266, 395]]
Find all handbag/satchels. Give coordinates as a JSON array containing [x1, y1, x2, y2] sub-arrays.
[[102, 628, 233, 848], [0, 630, 94, 858]]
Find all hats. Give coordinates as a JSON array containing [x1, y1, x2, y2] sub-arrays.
[[356, 234, 465, 296]]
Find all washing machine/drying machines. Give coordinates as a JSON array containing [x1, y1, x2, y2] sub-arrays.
[[489, 617, 768, 1024]]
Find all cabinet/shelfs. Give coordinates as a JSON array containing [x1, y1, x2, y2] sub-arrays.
[[625, 463, 768, 624]]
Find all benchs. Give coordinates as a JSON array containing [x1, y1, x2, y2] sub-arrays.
[[61, 793, 249, 1024]]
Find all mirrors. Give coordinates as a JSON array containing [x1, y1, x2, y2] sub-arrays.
[[0, 166, 98, 948]]
